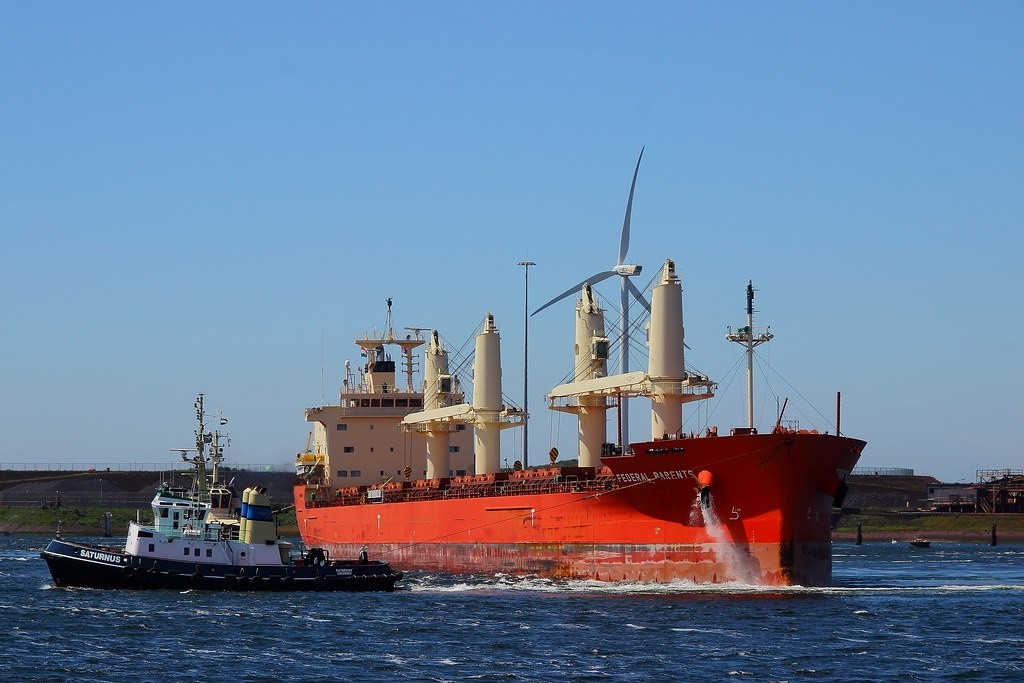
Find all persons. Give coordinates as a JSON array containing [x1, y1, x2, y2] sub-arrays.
[[310, 548, 325, 578]]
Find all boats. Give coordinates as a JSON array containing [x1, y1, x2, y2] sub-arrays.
[[293, 144, 867, 588], [38, 392, 404, 591], [909, 537, 932, 547]]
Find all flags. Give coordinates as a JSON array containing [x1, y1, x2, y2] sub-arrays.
[[220, 418, 228, 425]]
